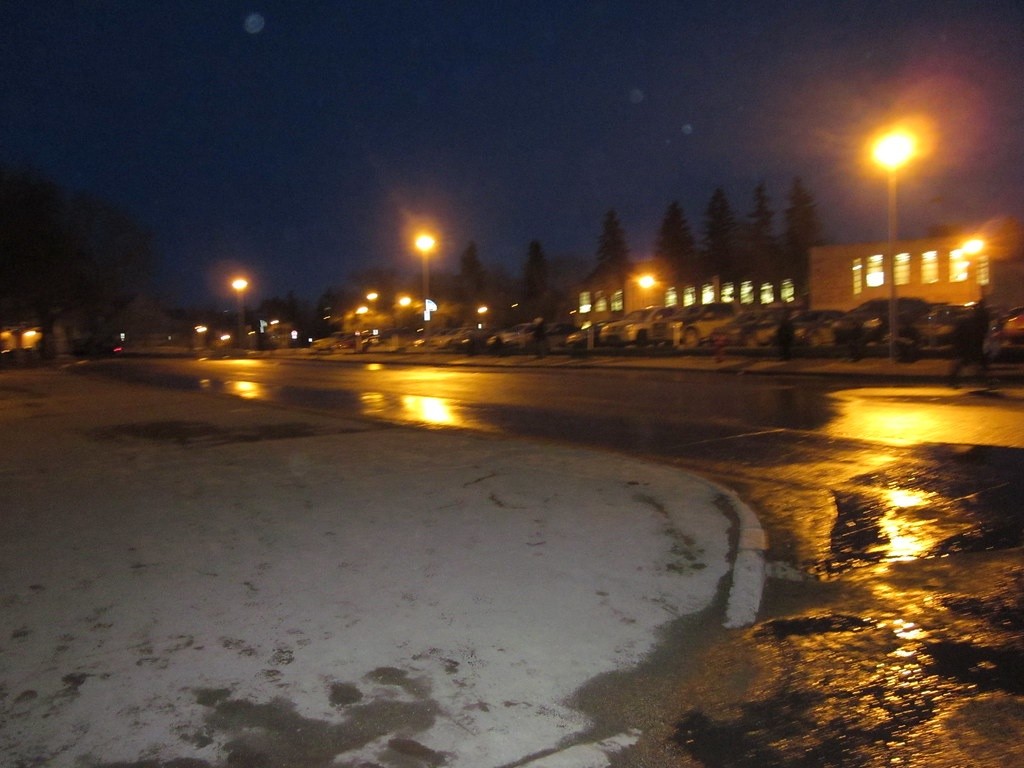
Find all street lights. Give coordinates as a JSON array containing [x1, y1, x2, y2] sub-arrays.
[[871, 129, 910, 363], [416, 233, 435, 348]]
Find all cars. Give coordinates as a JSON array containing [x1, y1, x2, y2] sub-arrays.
[[307, 297, 1024, 358]]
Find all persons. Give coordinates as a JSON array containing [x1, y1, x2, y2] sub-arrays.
[[532, 317, 545, 359], [777, 311, 793, 361], [39, 328, 80, 368], [945, 299, 1000, 386]]
